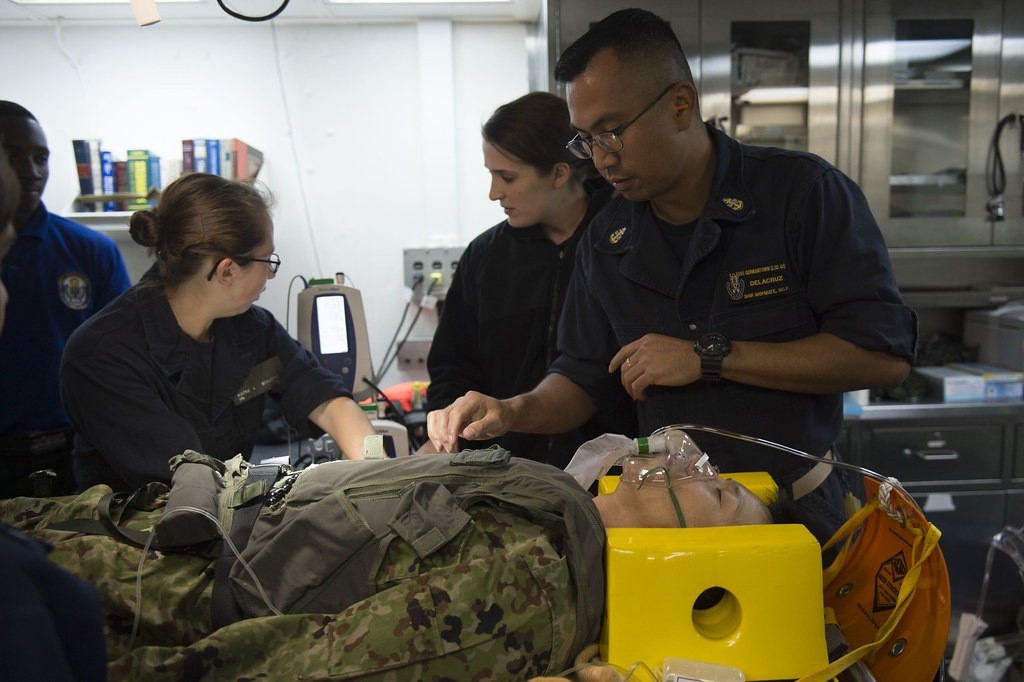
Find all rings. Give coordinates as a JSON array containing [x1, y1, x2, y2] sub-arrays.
[[626, 358, 633, 368]]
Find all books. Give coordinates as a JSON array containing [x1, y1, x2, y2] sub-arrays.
[[66, 136, 266, 210]]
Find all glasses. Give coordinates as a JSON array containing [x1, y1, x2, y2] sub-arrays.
[[569, 83, 681, 160], [205, 255, 281, 282]]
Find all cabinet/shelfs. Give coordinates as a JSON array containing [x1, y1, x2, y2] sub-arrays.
[[525, 1, 1024, 304], [849, 407, 1024, 638]]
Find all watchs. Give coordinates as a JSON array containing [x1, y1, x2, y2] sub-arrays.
[[694, 332, 734, 381]]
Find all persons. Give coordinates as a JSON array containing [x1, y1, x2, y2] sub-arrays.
[[0, 454, 865, 682], [58, 173, 391, 490], [0, 99, 135, 496], [426, 7, 920, 509], [426, 91, 640, 468]]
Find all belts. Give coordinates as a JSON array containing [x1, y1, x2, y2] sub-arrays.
[[206, 460, 289, 626]]
